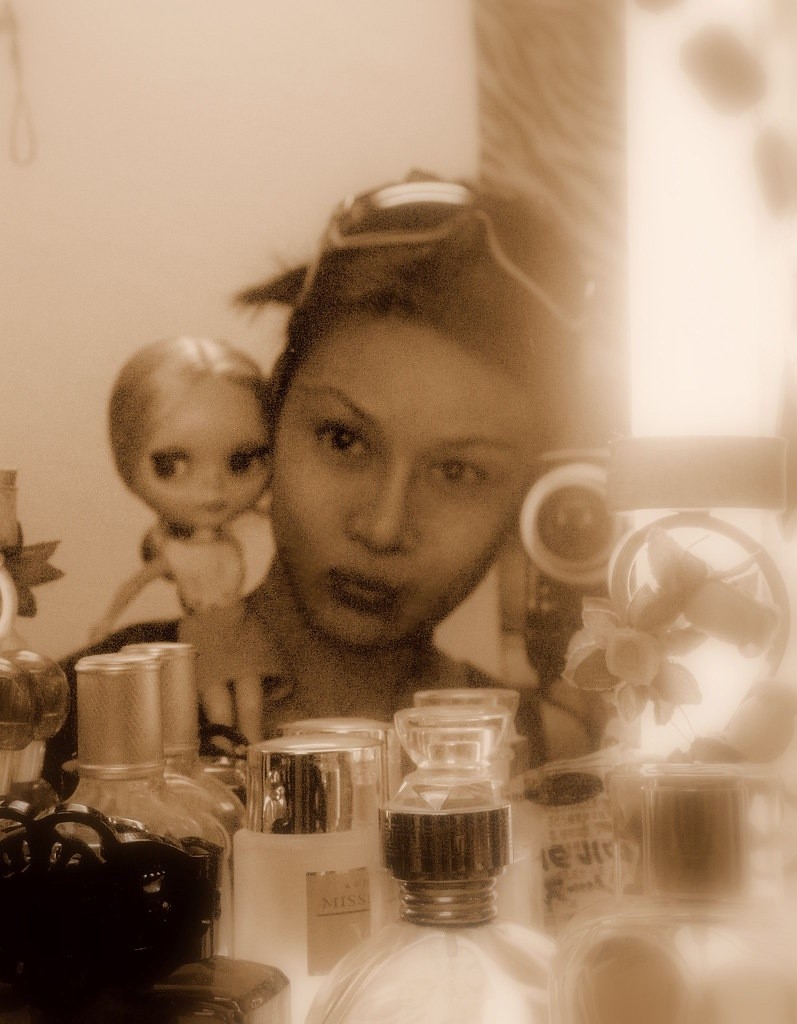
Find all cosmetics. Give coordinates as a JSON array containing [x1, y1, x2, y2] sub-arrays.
[[0, 633, 796, 1023]]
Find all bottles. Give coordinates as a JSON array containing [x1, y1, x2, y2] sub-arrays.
[[550, 769, 797, 1024], [53, 654, 230, 959], [119, 641, 246, 856], [307, 703, 558, 1024]]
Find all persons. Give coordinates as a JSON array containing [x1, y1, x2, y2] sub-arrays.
[[79, 338, 299, 761], [51, 174, 605, 808]]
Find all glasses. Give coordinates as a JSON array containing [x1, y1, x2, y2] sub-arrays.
[[296, 180, 584, 344]]
